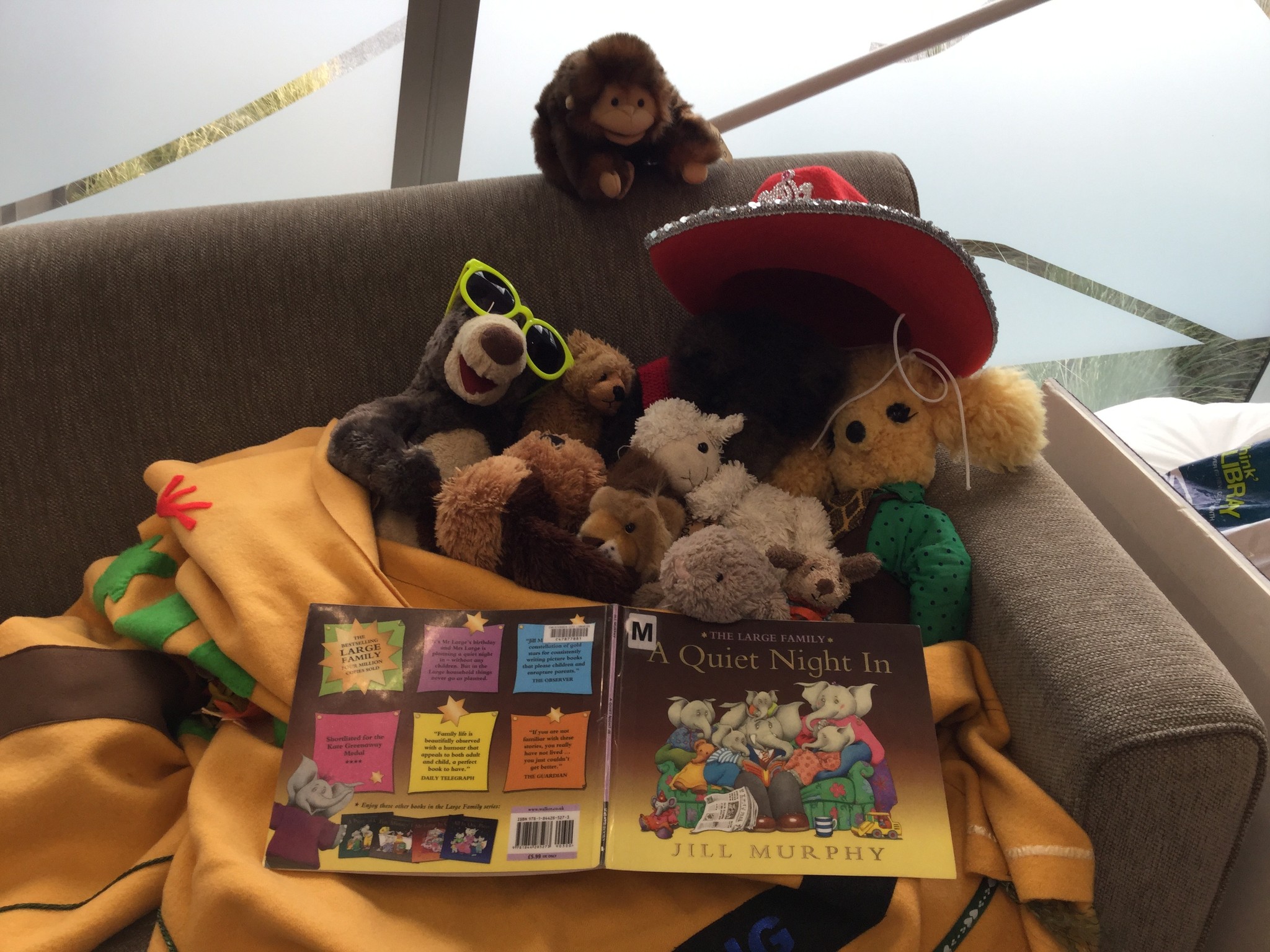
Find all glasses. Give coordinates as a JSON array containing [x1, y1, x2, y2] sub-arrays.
[[441, 256, 577, 382]]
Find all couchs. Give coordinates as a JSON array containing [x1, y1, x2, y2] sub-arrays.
[[1, 150, 1265, 950]]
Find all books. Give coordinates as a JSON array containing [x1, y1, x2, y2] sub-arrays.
[[262, 599, 961, 882]]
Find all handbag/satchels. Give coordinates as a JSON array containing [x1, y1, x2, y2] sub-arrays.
[[1094, 395, 1269, 584]]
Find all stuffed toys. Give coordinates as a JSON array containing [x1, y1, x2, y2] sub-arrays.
[[652, 521, 797, 624], [630, 395, 878, 614], [643, 260, 848, 480], [530, 28, 724, 204], [433, 427, 631, 607], [781, 338, 1056, 673], [324, 286, 532, 531], [569, 481, 685, 609], [532, 327, 641, 463]]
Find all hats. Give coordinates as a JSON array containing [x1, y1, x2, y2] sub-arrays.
[[647, 168, 1003, 382]]
